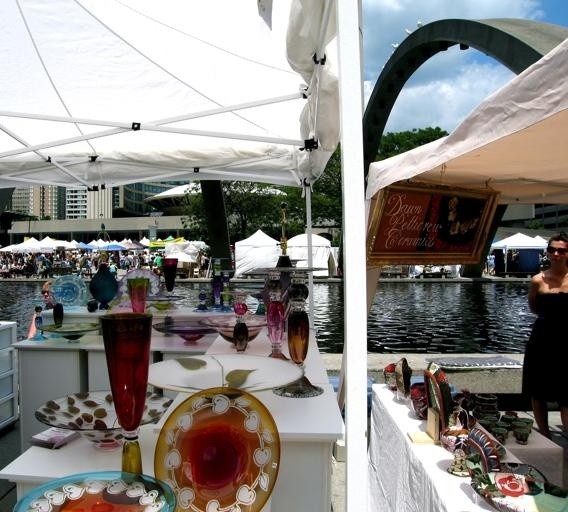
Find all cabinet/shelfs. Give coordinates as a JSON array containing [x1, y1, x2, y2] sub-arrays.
[[0, 319, 18, 427]]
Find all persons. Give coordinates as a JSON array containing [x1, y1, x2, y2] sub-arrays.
[[28, 279, 50, 339], [521, 235, 568, 440], [0, 249, 164, 279]]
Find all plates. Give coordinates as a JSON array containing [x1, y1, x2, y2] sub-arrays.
[[466, 428, 502, 479], [154, 387, 281, 512], [148, 353, 304, 393], [118, 269, 162, 297], [153, 321, 218, 345], [427, 361, 453, 430], [13, 471, 177, 512], [198, 315, 268, 348], [471, 472, 568, 511], [470, 462, 549, 483], [35, 390, 174, 453], [491, 439, 506, 459], [395, 358, 410, 399], [38, 323, 102, 343], [49, 275, 88, 311]]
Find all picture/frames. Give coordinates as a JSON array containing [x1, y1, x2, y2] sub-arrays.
[[362, 180, 501, 265]]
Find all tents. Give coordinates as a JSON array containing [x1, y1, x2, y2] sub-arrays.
[[286, 232, 330, 277], [0, 0, 371, 511], [362, 34, 568, 213], [535, 235, 546, 242], [491, 232, 547, 275], [233, 229, 282, 278], [0, 236, 151, 255]]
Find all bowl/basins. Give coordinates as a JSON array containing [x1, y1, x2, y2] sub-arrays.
[[383, 363, 397, 391]]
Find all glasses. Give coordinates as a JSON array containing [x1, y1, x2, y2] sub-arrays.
[[547, 245, 567, 254], [41, 290, 50, 294]]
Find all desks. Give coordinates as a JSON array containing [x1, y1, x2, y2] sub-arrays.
[[469, 410, 563, 489], [13, 308, 344, 511], [1, 393, 177, 505]]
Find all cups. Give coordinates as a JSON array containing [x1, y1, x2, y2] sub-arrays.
[[477, 420, 492, 432], [160, 258, 178, 292], [127, 278, 150, 313], [512, 421, 527, 431], [501, 415, 516, 431], [484, 416, 498, 428], [516, 418, 534, 433], [514, 428, 529, 445], [495, 422, 511, 433], [492, 428, 508, 446]]
